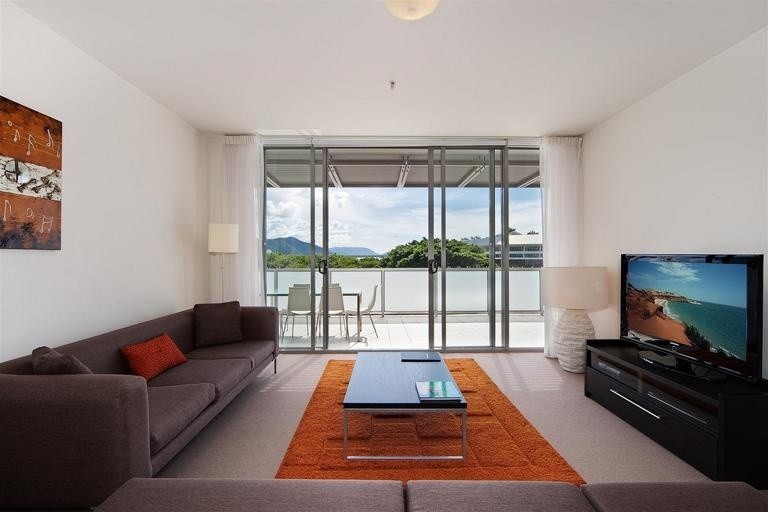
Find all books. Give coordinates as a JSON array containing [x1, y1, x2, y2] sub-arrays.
[[415, 381, 462, 401]]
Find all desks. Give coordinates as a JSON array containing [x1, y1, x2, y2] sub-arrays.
[[267, 289, 363, 343]]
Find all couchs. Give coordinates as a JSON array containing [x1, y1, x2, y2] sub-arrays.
[[88, 473, 767, 511], [0, 297, 280, 512]]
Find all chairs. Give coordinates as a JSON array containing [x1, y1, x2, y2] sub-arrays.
[[282, 286, 313, 342], [321, 283, 343, 339], [315, 286, 348, 340], [345, 281, 380, 341], [291, 280, 312, 339]]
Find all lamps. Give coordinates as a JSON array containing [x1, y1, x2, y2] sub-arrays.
[[206, 223, 241, 304], [538, 266, 610, 374], [384, 0, 440, 21]]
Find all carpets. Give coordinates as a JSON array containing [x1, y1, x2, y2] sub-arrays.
[[273, 358, 587, 487]]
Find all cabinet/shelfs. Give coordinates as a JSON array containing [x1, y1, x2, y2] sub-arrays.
[[584, 337, 768, 483]]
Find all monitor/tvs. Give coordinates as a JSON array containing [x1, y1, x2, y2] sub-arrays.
[[618, 253, 764, 384]]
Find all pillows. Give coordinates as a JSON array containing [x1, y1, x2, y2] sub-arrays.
[[28, 345, 97, 376], [192, 301, 245, 349], [115, 331, 190, 382]]
